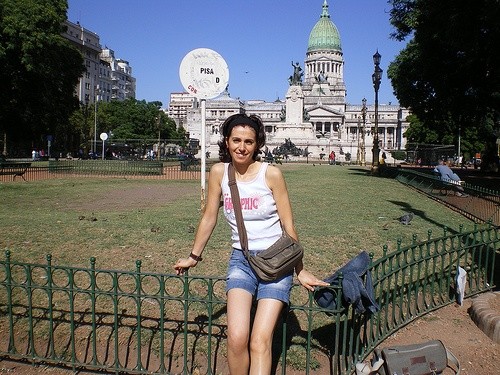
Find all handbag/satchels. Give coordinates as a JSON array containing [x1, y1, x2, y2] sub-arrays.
[[373, 339, 448, 375], [248, 230, 304, 282]]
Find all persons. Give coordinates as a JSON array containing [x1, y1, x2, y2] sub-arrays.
[[67, 147, 201, 165], [434, 158, 468, 198], [318, 69, 324, 80], [173, 113, 330, 375], [32, 148, 45, 160], [328, 150, 336, 165], [380, 157, 386, 166], [290, 60, 302, 77]]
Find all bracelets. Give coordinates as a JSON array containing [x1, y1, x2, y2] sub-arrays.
[[190, 252, 203, 261]]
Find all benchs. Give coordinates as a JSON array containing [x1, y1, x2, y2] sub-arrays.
[[431, 172, 457, 196], [48, 160, 74, 174], [0, 163, 32, 181]]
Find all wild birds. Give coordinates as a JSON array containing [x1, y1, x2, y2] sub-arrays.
[[185, 221, 196, 234], [77, 211, 97, 224], [381, 221, 391, 230], [149, 226, 161, 233]]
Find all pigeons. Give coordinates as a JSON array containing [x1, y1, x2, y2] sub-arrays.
[[319, 131, 327, 137], [392, 212, 415, 227], [245, 71, 248, 74], [312, 163, 316, 167]]
